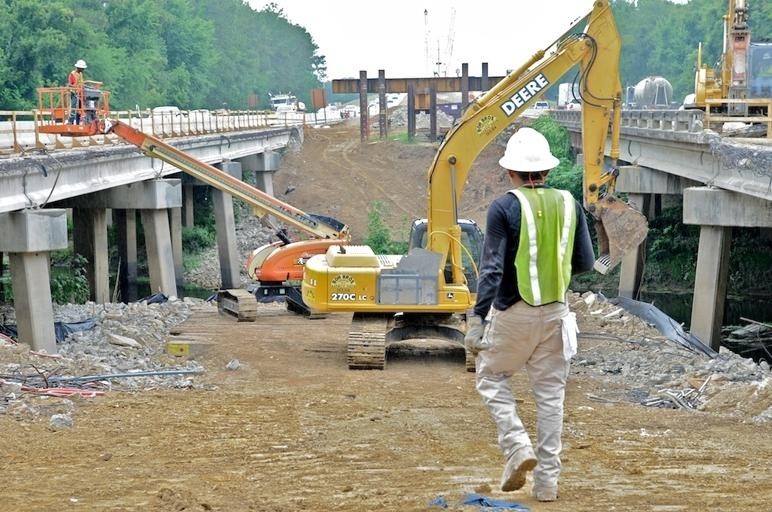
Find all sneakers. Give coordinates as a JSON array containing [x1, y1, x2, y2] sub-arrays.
[[531, 483, 557, 502], [500, 447, 537, 491]]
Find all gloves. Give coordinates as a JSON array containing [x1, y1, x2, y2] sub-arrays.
[[464, 317, 490, 356]]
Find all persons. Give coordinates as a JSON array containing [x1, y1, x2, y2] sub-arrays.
[[463, 128, 596, 501], [67, 58, 88, 124]]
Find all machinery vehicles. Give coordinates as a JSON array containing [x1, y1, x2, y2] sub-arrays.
[[685, 0, 771, 130], [34, 60, 351, 323], [300, 0, 650, 371]]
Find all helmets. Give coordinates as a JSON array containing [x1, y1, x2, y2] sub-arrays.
[[498, 128, 560, 172], [75, 60, 87, 68]]
